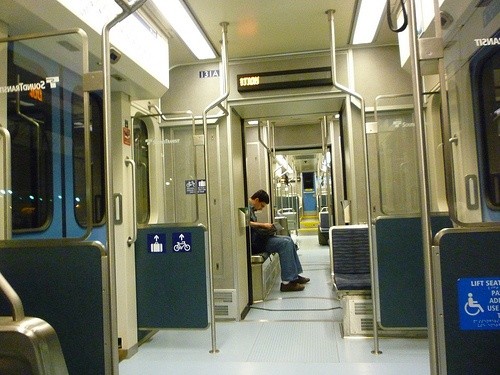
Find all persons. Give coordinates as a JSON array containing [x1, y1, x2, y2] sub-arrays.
[[248, 189, 311, 291]]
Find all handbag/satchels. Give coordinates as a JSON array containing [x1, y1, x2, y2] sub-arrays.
[[251, 228, 266, 254]]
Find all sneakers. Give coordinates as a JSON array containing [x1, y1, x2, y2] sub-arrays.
[[290, 275, 310, 284], [281, 281, 305, 291]]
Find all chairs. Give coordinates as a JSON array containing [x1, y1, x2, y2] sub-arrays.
[[319, 211, 330, 233], [328, 226, 372, 289]]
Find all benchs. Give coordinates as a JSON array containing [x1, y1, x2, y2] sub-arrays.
[[252, 251, 279, 301]]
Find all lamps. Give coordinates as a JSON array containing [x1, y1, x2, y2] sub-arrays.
[[348, 0, 387, 45], [152, 0, 219, 62]]
